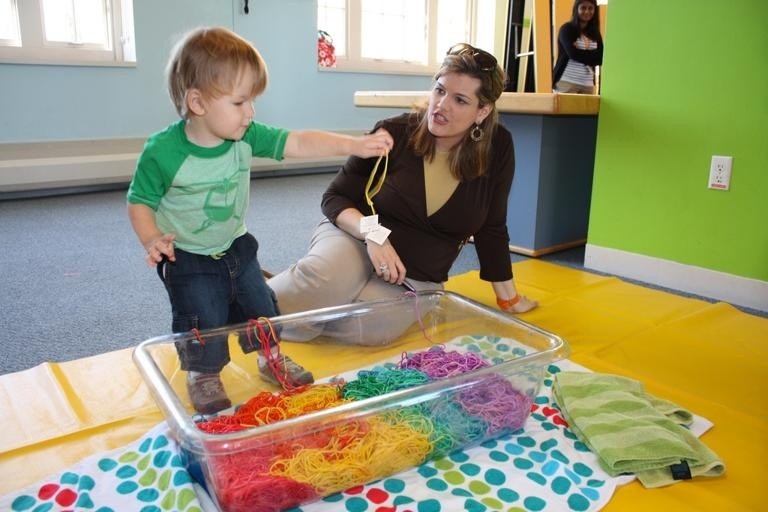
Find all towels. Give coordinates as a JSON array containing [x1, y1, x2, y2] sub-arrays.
[[550, 369, 729, 489]]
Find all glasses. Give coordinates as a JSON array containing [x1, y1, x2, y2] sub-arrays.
[[447, 42, 497, 92]]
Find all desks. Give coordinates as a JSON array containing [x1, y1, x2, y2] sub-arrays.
[[355, 86, 600, 263]]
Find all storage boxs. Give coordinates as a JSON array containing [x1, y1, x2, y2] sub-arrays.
[[133, 287, 563, 512]]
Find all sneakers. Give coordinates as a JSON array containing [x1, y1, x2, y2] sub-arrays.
[[256, 352, 314, 389], [186, 369, 231, 413]]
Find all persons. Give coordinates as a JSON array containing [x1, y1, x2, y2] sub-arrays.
[[125, 25, 394, 414], [260, 43, 540, 345], [552, 0, 603, 94]]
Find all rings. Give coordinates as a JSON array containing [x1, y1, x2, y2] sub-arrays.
[[379, 264, 389, 272]]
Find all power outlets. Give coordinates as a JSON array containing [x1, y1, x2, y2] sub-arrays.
[[706, 154, 735, 192]]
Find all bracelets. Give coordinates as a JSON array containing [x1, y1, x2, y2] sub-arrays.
[[494, 292, 522, 310]]
